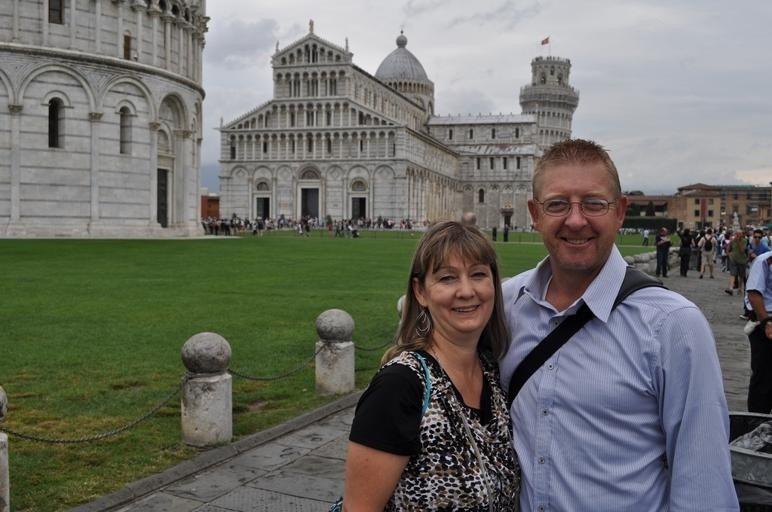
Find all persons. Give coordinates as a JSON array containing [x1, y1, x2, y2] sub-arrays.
[[499, 140, 741, 512], [642, 222, 772, 414], [492, 227, 496, 241], [201, 214, 414, 238], [503, 224, 509, 242], [329, 221, 521, 512]]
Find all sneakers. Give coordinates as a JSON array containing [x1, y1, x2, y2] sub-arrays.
[[656, 274, 667, 277], [700, 275, 714, 278], [725, 290, 732, 295], [740, 315, 749, 319]]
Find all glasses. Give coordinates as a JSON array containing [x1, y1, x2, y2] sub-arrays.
[[754, 236, 761, 239], [536, 198, 616, 216]]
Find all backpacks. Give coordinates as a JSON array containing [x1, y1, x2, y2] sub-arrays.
[[704, 236, 712, 250]]
